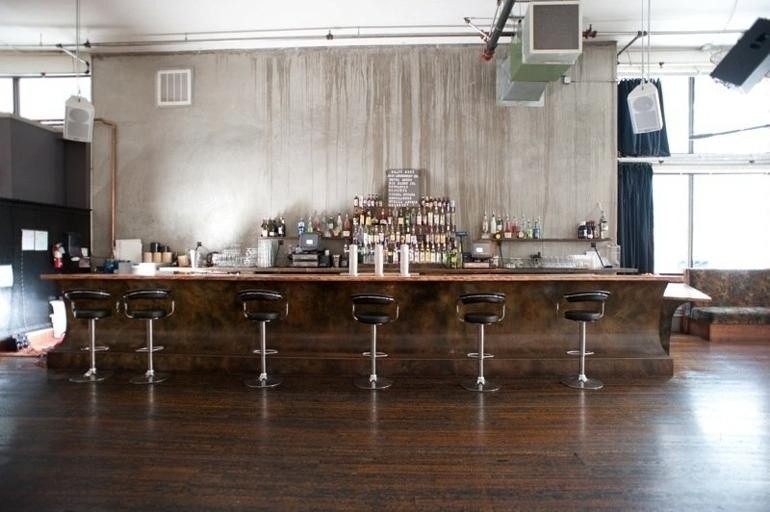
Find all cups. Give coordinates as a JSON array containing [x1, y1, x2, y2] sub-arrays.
[[178, 255, 189, 267], [145, 242, 173, 263], [333, 254, 341, 268], [118, 262, 132, 274], [216, 248, 257, 268]]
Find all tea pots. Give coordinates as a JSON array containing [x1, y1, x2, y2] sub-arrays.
[[207, 252, 219, 266]]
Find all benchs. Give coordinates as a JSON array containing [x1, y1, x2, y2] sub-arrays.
[[683, 265, 769, 345]]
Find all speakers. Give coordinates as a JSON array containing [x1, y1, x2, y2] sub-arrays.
[[626, 82, 663, 135], [709, 17, 770, 94], [63, 96, 95, 143]]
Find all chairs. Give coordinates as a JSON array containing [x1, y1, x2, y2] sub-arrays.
[[453, 288, 511, 393], [233, 287, 296, 391], [120, 288, 179, 390], [56, 290, 122, 390], [346, 290, 402, 391], [554, 288, 609, 393]]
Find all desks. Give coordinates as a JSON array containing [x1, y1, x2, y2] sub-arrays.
[[659, 282, 711, 356]]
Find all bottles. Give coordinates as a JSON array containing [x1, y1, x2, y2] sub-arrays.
[[194, 242, 204, 251], [481, 211, 541, 240], [421, 196, 450, 208], [297, 212, 351, 239], [576, 220, 599, 239], [598, 211, 608, 240], [353, 192, 383, 209], [261, 217, 287, 238], [350, 206, 462, 270]]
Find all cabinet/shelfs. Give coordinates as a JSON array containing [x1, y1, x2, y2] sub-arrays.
[[351, 202, 458, 270]]
